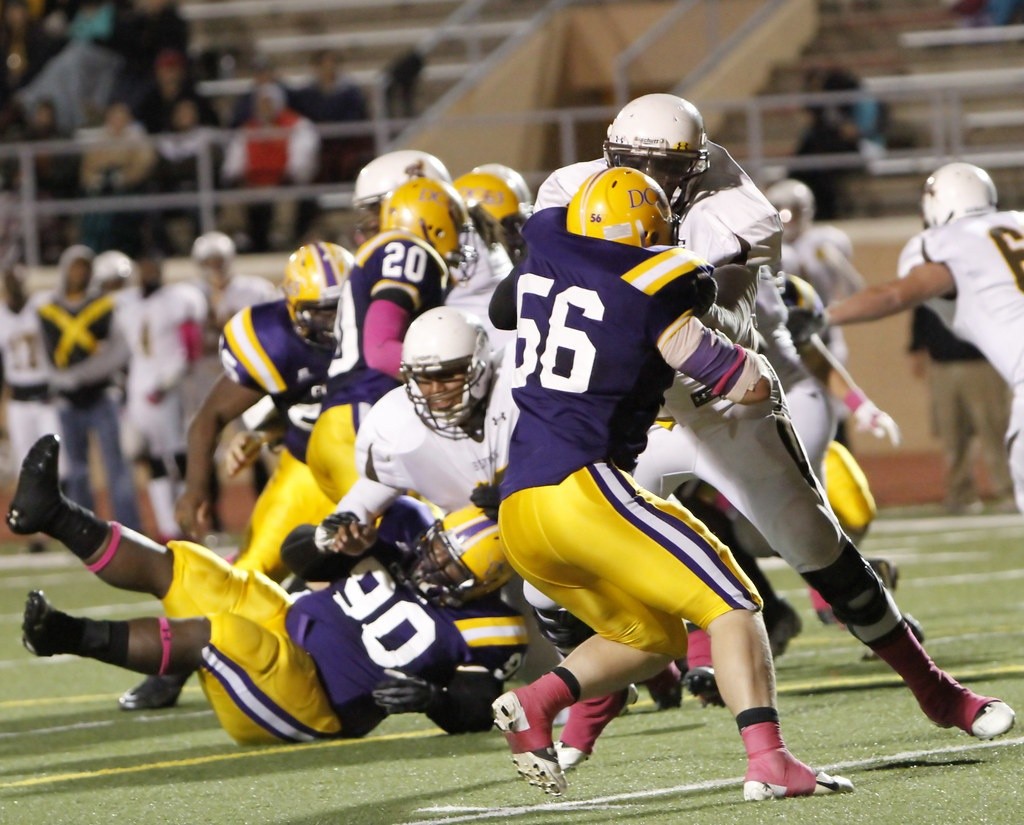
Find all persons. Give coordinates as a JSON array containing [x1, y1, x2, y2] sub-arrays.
[[784, 47, 885, 219], [1, 91, 1023, 804], [0, 0, 374, 257], [951, 0, 1024, 31]]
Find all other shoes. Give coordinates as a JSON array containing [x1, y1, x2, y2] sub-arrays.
[[766, 600, 802, 657], [119, 675, 187, 710]]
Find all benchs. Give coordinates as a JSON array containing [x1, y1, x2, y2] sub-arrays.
[[0, 0, 557, 281], [709, 0, 1024, 213]]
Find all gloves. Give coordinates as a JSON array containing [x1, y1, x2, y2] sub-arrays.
[[373, 669, 434, 713], [852, 399, 902, 446]]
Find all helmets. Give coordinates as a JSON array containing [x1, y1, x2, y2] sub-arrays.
[[283, 242, 353, 341], [920, 163, 997, 229], [379, 178, 479, 282], [764, 178, 815, 241], [472, 163, 533, 213], [602, 93, 711, 213], [452, 173, 520, 221], [566, 167, 680, 248], [353, 150, 452, 211], [401, 306, 492, 440], [54, 229, 241, 295], [408, 505, 515, 608]]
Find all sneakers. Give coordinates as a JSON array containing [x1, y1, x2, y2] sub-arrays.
[[492, 691, 567, 797], [742, 751, 853, 802], [684, 664, 725, 708], [557, 685, 639, 771], [920, 673, 1016, 740], [6, 434, 63, 534], [22, 590, 56, 656]]
